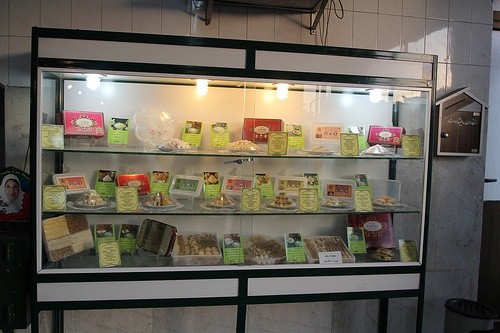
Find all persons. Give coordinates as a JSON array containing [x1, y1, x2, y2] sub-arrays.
[[0, 174, 30, 221]]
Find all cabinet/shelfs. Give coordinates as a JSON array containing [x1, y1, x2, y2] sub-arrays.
[[30, 27, 439, 333]]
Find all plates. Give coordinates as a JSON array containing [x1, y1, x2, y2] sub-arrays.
[[206, 203, 239, 208], [320, 205, 351, 209], [155, 147, 191, 152], [370, 255, 398, 261], [142, 203, 178, 208], [226, 148, 260, 154], [73, 203, 111, 208], [372, 202, 401, 209], [362, 151, 393, 156], [303, 150, 338, 155], [269, 204, 296, 209]]
